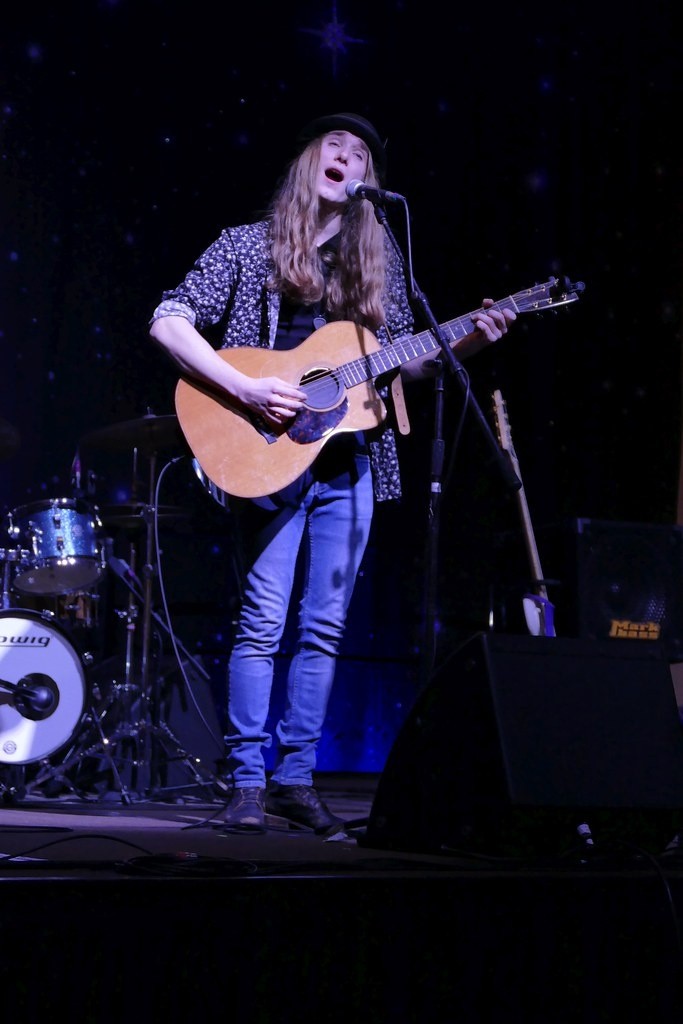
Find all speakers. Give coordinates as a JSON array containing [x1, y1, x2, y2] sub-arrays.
[[351, 628, 683, 868]]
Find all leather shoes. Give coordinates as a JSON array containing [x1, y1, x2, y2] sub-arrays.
[[224, 784, 348, 834]]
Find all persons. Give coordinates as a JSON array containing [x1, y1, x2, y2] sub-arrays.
[[143, 106, 516, 835]]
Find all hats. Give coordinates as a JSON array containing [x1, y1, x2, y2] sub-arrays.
[[299, 114, 385, 170]]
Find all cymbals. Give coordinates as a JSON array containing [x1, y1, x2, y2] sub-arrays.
[[99, 514, 193, 532], [97, 500, 192, 511], [94, 415, 187, 451]]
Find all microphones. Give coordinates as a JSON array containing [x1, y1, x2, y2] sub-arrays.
[[345, 179, 406, 206]]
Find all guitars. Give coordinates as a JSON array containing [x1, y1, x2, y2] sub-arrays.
[[170, 275, 587, 499], [489, 387, 560, 638]]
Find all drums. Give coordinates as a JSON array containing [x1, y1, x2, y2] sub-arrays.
[[0, 607, 90, 766], [3, 495, 105, 590]]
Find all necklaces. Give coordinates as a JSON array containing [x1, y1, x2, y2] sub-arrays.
[[307, 303, 330, 328]]
